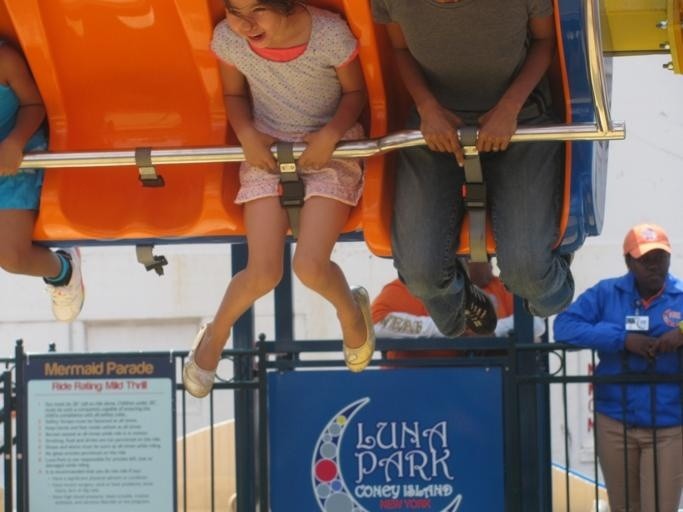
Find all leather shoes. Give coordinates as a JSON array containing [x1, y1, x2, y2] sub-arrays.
[[342, 287, 376, 373], [183, 322, 231, 398]]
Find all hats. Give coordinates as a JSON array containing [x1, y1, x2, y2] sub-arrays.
[[624, 224, 672, 258]]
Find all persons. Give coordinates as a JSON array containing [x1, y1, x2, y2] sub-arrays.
[[545, 219, 682, 512], [365, 1, 576, 340], [178, 1, 376, 402], [0, 40, 85, 323]]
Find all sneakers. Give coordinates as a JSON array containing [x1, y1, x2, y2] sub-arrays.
[[456, 258, 497, 334], [44, 246, 83, 322]]
